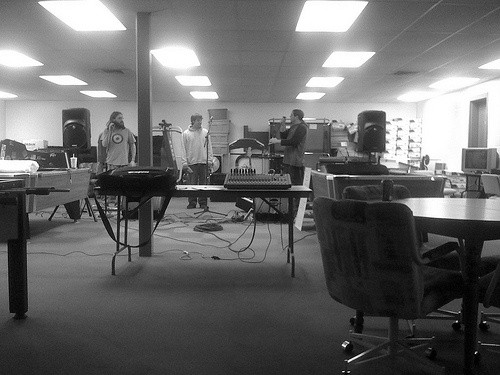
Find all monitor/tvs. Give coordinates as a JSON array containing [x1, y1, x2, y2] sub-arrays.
[[461, 147, 498, 172]]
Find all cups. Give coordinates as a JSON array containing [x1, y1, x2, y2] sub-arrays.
[[70, 158, 77, 169]]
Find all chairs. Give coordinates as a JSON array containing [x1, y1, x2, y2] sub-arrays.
[[311, 185, 500, 375]]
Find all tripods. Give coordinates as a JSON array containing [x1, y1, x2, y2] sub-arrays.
[[193, 121, 227, 221]]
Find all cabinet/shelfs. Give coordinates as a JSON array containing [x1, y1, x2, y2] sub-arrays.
[[269, 121, 330, 170]]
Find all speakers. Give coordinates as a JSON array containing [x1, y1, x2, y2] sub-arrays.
[[62, 108, 92, 151], [358, 111, 387, 153]]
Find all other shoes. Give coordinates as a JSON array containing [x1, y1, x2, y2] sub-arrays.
[[200, 204, 209, 208], [187, 203, 196, 209]]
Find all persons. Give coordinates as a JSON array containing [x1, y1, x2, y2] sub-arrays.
[[102, 111, 137, 208], [180, 113, 214, 208], [268, 109, 308, 224]]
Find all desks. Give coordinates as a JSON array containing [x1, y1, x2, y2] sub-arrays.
[[310, 171, 450, 203], [112, 184, 312, 279], [391, 197, 500, 375]]
